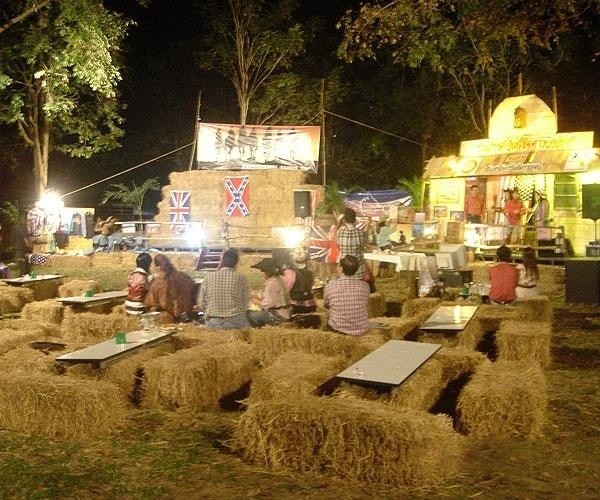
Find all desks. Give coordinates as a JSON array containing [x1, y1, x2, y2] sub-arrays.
[[363, 213, 565, 271], [337, 339, 443, 387], [56, 291, 129, 303], [2, 274, 64, 283], [419, 305, 480, 331], [54, 330, 178, 361]]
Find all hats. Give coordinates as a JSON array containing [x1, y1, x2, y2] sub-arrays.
[[291, 247, 310, 270], [250, 257, 280, 273]]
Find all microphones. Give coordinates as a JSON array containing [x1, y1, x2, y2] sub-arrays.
[[499, 196, 505, 201]]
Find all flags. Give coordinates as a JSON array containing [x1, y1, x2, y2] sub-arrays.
[[224, 176, 251, 215], [308, 225, 343, 265], [169, 191, 190, 232]]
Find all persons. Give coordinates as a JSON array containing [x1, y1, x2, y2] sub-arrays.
[[244, 246, 317, 327], [399, 231, 407, 246], [514, 246, 540, 298], [464, 185, 488, 226], [144, 254, 203, 324], [488, 246, 519, 304], [124, 252, 153, 319], [376, 216, 398, 278], [92, 216, 120, 255], [489, 187, 556, 242], [331, 209, 376, 298], [193, 249, 254, 330], [323, 256, 373, 336]]
